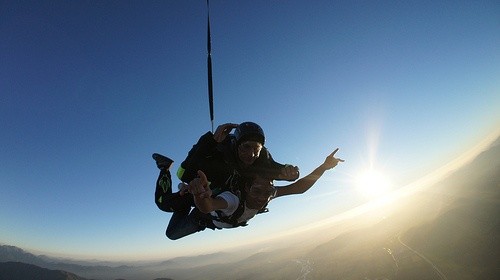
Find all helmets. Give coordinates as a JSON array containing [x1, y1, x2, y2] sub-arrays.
[[234, 122, 264, 146], [243, 176, 277, 193]]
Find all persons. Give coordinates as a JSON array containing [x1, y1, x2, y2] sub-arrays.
[[165, 148, 345, 240], [152, 122, 300, 213]]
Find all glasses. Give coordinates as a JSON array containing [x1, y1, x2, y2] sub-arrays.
[[239, 144, 262, 152]]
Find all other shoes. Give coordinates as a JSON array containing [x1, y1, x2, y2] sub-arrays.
[[152, 153, 174, 169], [178, 183, 186, 189]]
